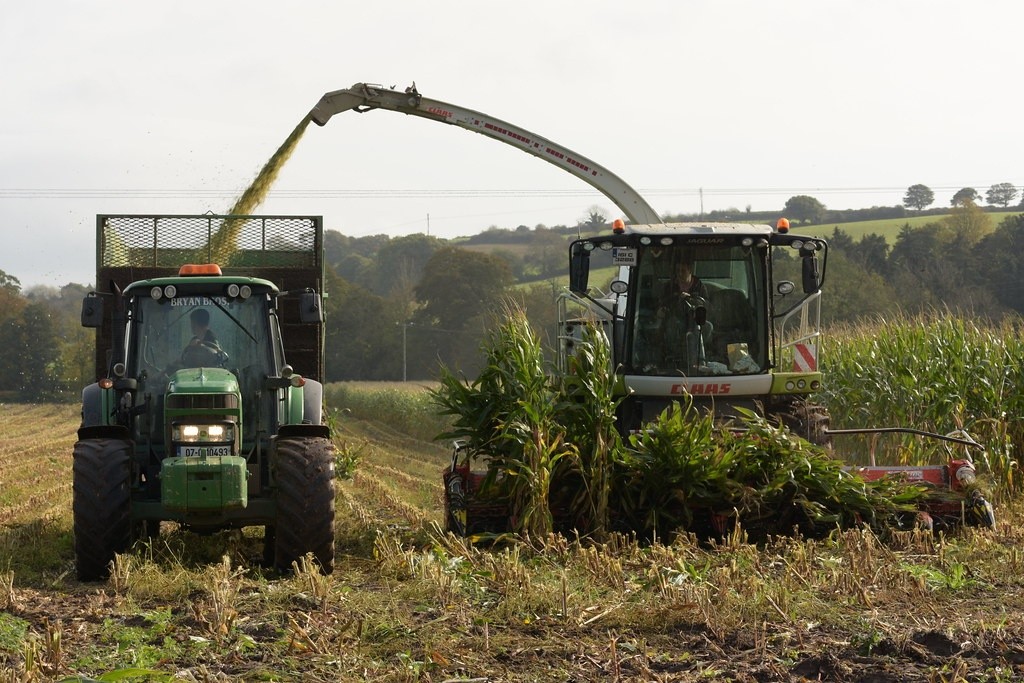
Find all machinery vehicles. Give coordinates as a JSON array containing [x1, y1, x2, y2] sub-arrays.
[[309, 80, 996, 539]]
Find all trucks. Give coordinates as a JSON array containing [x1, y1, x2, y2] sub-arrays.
[[73, 208, 336, 579]]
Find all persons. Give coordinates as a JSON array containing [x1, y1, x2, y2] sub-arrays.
[[660, 256, 714, 349], [180, 310, 218, 363]]
